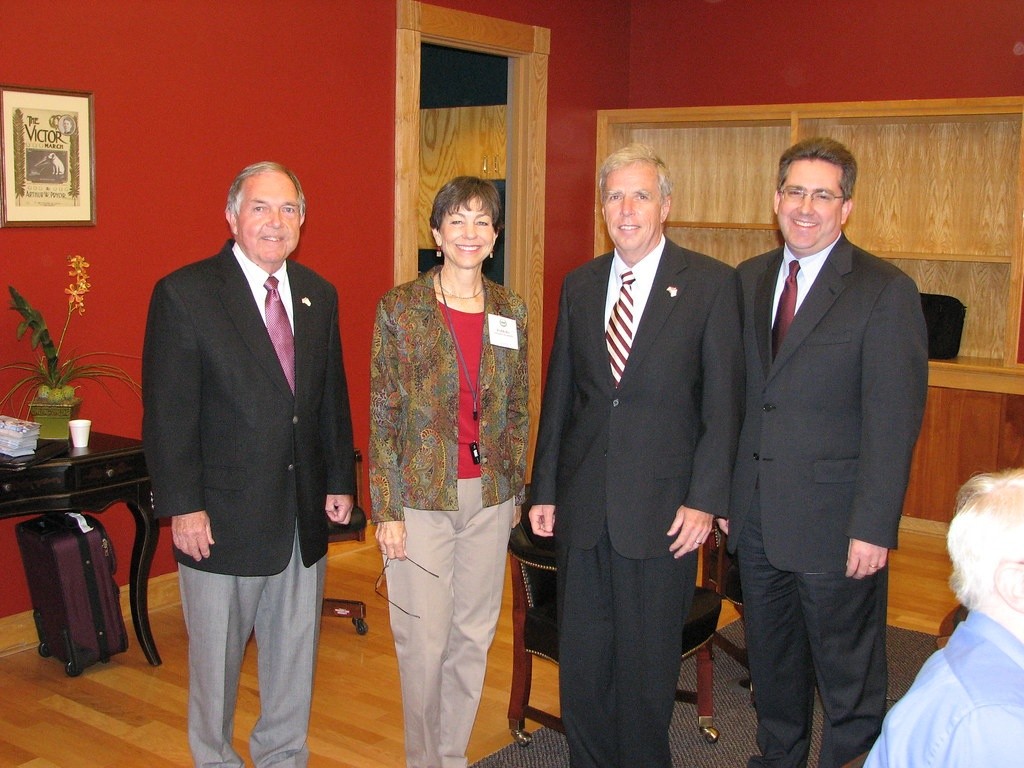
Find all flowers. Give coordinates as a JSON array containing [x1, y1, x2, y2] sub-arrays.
[[0, 254, 142, 420]]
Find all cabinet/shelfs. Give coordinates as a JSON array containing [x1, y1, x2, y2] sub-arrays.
[[591, 96, 1023, 396]]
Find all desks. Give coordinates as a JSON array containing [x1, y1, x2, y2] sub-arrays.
[[0, 431, 162, 667]]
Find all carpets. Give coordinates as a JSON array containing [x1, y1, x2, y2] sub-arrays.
[[468, 615, 937, 768]]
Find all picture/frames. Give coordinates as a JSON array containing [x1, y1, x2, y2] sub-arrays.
[[0, 83, 98, 230]]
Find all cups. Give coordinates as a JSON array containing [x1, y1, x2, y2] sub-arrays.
[[69, 419, 91, 447]]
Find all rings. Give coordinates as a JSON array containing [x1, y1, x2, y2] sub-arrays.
[[695, 541, 701, 544], [869, 564, 878, 568]]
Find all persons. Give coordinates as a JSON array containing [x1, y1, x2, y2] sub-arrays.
[[528, 144, 746, 768], [860, 469, 1024, 768], [364, 176, 532, 768], [723, 137, 928, 768], [137, 160, 356, 768]]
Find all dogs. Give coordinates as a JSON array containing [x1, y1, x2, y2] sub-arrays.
[[48, 153, 66, 176]]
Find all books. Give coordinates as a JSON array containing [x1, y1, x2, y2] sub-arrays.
[[0, 414, 41, 458]]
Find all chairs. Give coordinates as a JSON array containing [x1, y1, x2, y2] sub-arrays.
[[507, 483, 719, 746], [321, 450, 369, 635]]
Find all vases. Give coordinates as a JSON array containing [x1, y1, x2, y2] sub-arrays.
[[28, 397, 83, 439]]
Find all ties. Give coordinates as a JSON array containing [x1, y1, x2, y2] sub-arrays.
[[771, 260, 801, 362], [262, 276, 297, 399], [605, 270, 636, 389]]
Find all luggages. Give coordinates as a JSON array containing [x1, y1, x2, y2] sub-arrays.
[[15, 512, 132, 676]]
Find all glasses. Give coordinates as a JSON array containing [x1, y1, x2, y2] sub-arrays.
[[776, 185, 843, 208]]
